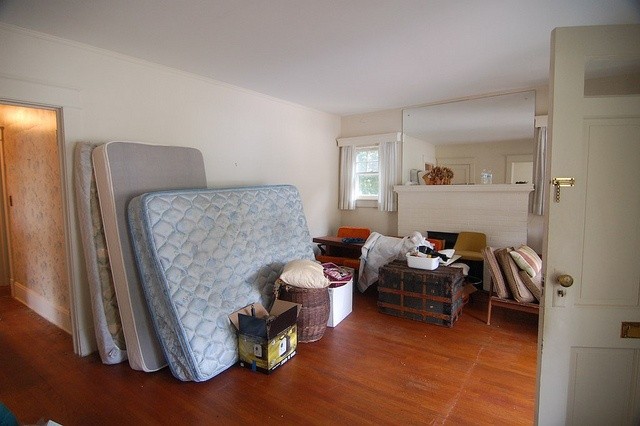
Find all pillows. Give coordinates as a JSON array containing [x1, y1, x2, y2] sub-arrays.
[[281, 259, 331, 289], [481, 246, 511, 299], [496, 247, 536, 303], [509, 244, 542, 278], [519, 270, 542, 302]]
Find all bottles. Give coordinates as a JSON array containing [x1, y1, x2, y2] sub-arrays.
[[481, 169, 487, 185], [488, 170, 492, 184]]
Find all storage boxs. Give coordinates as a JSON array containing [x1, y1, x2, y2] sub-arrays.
[[325, 277, 353, 328], [228, 298, 302, 375], [376, 259, 463, 328]]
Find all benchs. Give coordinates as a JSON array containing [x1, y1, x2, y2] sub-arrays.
[[485, 254, 542, 326]]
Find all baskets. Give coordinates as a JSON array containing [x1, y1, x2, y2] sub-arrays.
[[321, 262, 352, 288], [280, 284, 330, 344]]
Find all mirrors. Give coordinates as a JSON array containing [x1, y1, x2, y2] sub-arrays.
[[402, 90, 535, 184]]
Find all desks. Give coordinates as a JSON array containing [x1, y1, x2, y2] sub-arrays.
[[313, 235, 364, 257]]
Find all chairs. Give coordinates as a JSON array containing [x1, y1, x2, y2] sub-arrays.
[[453, 231, 486, 261], [316, 226, 371, 268]]
